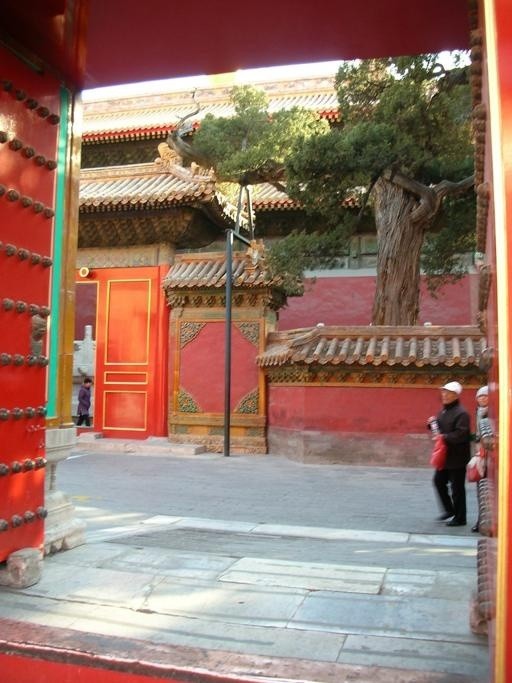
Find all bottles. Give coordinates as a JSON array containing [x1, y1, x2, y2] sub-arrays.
[[430, 420, 441, 437]]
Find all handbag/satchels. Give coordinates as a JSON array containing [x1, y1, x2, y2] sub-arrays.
[[431, 434, 447, 470], [466, 448, 486, 482]]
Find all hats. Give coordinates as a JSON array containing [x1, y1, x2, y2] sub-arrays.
[[475, 385, 488, 398], [439, 381, 463, 395]]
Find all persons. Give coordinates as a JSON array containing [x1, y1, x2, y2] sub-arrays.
[[74, 378, 94, 427], [423, 379, 469, 527], [465, 384, 496, 538]]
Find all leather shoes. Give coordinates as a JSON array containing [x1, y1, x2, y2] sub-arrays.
[[440, 512, 479, 532]]
